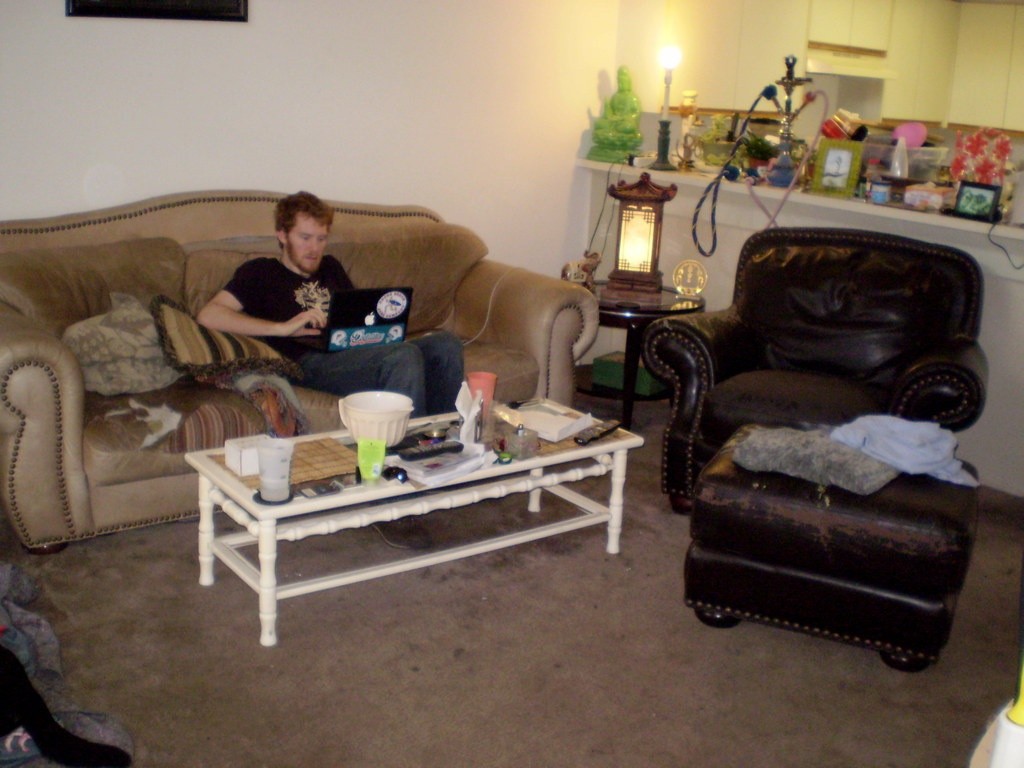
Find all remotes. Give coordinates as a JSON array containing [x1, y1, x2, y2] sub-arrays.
[[399, 441, 464, 462], [573, 420, 621, 446]]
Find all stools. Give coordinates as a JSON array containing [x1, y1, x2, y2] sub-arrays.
[[683, 423, 982, 674]]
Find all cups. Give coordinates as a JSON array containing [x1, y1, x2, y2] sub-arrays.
[[256, 437, 293, 501], [468, 370, 497, 418]]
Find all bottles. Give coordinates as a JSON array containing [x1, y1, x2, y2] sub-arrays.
[[890, 137, 908, 179], [864, 158, 880, 191], [871, 180, 891, 204]]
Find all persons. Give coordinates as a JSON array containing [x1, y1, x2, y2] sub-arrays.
[[195, 192, 512, 550]]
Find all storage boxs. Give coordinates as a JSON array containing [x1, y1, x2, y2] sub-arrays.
[[903, 183, 956, 216], [594, 351, 665, 395], [224, 433, 271, 478]]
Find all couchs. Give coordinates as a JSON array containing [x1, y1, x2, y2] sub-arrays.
[[641, 227, 989, 518], [0, 189, 598, 556]]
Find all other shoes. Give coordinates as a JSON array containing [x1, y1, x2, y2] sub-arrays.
[[375, 519, 432, 549]]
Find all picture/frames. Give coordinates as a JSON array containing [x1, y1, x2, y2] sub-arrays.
[[952, 179, 1002, 223], [807, 137, 864, 201]]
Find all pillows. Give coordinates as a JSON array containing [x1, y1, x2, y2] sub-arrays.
[[151, 294, 306, 392], [59, 310, 188, 395]]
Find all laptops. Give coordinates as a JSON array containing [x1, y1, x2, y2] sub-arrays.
[[309, 286, 413, 353]]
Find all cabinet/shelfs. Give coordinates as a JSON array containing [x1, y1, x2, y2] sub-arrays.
[[806, 0, 1024, 136]]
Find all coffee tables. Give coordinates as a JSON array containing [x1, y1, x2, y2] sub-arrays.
[[183, 400, 644, 648], [577, 280, 706, 432]]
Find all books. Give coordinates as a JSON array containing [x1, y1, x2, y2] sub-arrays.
[[385, 443, 486, 485]]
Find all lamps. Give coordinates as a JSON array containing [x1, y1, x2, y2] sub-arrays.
[[646, 46, 679, 171], [606, 172, 678, 294]]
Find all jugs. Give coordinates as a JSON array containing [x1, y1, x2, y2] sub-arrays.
[[340, 391, 415, 447]]
